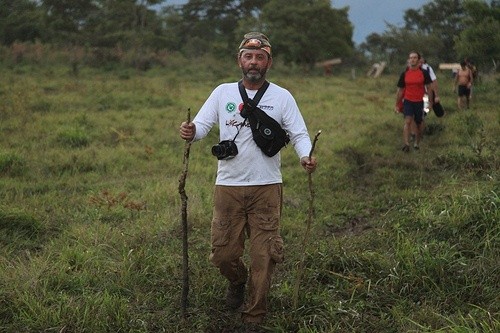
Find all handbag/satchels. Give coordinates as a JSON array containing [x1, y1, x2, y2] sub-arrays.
[[247, 107, 290, 157], [432, 102, 444, 118]]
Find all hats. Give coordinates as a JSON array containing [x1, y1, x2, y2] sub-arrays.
[[238, 31, 272, 59]]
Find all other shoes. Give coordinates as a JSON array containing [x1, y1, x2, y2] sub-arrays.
[[414, 144, 419, 149], [227, 284, 245, 309], [402, 143, 410, 152]]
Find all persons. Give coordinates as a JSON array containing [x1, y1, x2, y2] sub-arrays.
[[411, 53, 440, 138], [180, 32, 317, 333], [453, 61, 473, 112], [394, 50, 433, 152]]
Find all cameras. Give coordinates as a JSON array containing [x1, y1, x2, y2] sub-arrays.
[[211, 140, 238, 161]]
